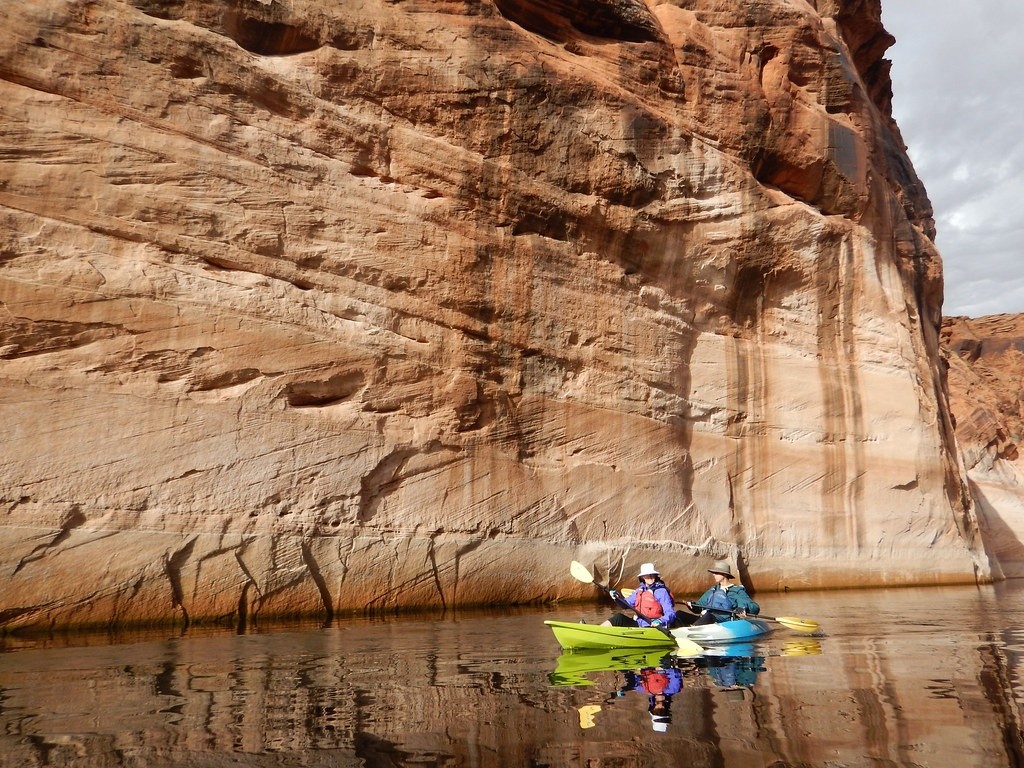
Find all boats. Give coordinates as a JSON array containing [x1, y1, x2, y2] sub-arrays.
[[544, 620, 679, 651], [669, 618, 776, 643]]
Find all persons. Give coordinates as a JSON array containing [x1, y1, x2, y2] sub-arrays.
[[580, 562, 676, 629], [670, 561, 760, 628], [616, 654, 683, 732], [695, 655, 766, 700]]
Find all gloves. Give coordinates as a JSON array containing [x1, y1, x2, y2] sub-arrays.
[[609, 590, 618, 599], [650, 619, 662, 628]]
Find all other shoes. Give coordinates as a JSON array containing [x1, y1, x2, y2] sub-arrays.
[[579, 619, 586, 624]]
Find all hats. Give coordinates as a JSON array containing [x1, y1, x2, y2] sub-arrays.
[[649, 711, 673, 732], [637, 563, 660, 576], [708, 561, 735, 579], [719, 689, 747, 702]]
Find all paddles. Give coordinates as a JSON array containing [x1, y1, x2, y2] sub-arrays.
[[620, 587, 820, 633], [570, 560, 706, 652]]
